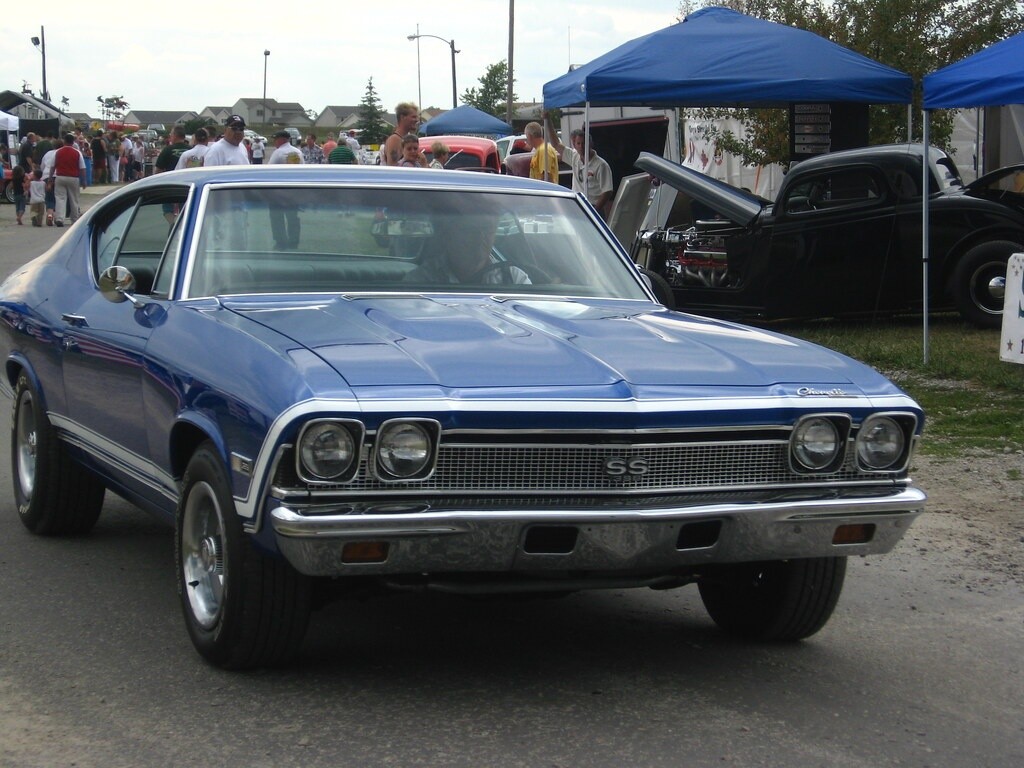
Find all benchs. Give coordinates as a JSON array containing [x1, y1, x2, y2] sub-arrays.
[[123, 258, 418, 295]]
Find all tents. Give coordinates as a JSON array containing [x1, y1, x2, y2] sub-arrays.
[[420, 105, 514, 138], [538, 6, 920, 365], [913, 33, 1024, 365]]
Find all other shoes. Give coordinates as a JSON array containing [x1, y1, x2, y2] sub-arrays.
[[56, 219, 64, 227], [46, 215, 53, 226], [32, 216, 38, 225]]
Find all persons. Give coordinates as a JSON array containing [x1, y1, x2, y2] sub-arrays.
[[0, 98, 452, 250], [542, 114, 613, 224], [404, 196, 533, 286], [522, 121, 561, 189]]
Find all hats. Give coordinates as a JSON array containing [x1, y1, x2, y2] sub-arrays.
[[117, 132, 128, 138], [131, 134, 139, 137], [337, 137, 346, 145], [20, 137, 27, 143], [224, 115, 245, 126], [271, 131, 290, 139], [349, 131, 354, 134]]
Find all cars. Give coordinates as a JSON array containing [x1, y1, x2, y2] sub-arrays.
[[365, 149, 380, 164], [635, 140, 1024, 325], [371, 135, 502, 246], [185, 135, 193, 144], [284, 128, 301, 145], [137, 124, 166, 141], [219, 130, 268, 145], [1, 164, 15, 202], [0, 164, 929, 667], [493, 132, 576, 178]]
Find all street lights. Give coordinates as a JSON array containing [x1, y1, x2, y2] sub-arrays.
[[32, 37, 47, 102], [262, 50, 271, 138], [408, 35, 458, 110]]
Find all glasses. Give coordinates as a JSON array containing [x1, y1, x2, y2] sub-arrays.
[[228, 127, 245, 131]]
